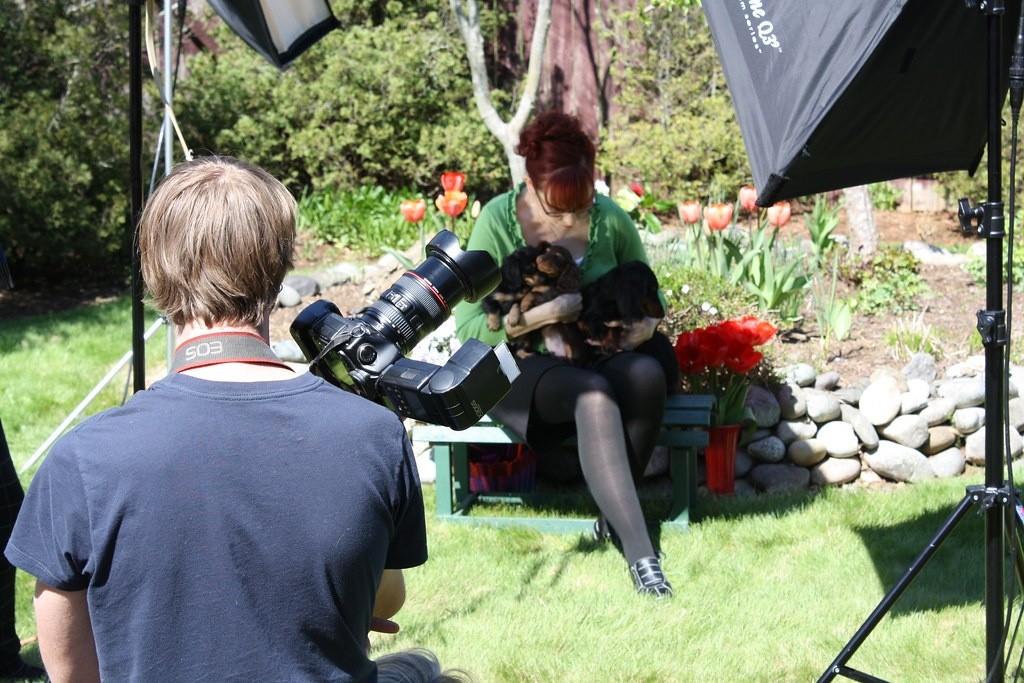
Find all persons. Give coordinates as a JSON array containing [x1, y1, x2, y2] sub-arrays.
[[454, 111, 676, 603], [0, 158, 470, 683]]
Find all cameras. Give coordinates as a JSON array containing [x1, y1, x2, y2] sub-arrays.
[[287, 227, 522, 430]]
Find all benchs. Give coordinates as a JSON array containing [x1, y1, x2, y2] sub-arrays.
[[406, 394, 719, 532]]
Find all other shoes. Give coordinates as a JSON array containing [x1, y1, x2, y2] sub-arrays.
[[594, 520, 614, 543], [631, 556, 673, 601]]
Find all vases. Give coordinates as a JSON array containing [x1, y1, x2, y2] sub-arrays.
[[705, 424, 744, 497]]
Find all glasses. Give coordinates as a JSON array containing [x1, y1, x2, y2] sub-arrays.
[[533, 184, 596, 218]]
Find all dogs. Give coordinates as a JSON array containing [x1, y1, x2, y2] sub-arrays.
[[480, 240, 664, 359]]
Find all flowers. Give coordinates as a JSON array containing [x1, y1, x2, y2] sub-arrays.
[[676, 313, 781, 449]]
